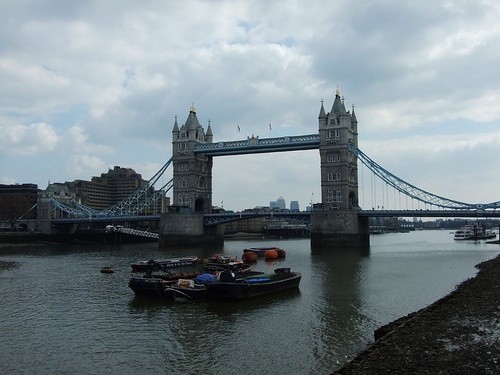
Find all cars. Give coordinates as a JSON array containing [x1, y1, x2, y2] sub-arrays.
[[104, 224, 125, 232], [119, 208, 313, 217]]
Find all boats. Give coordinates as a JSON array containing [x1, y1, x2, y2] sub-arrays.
[[127, 246, 303, 303], [453, 222, 497, 241]]
